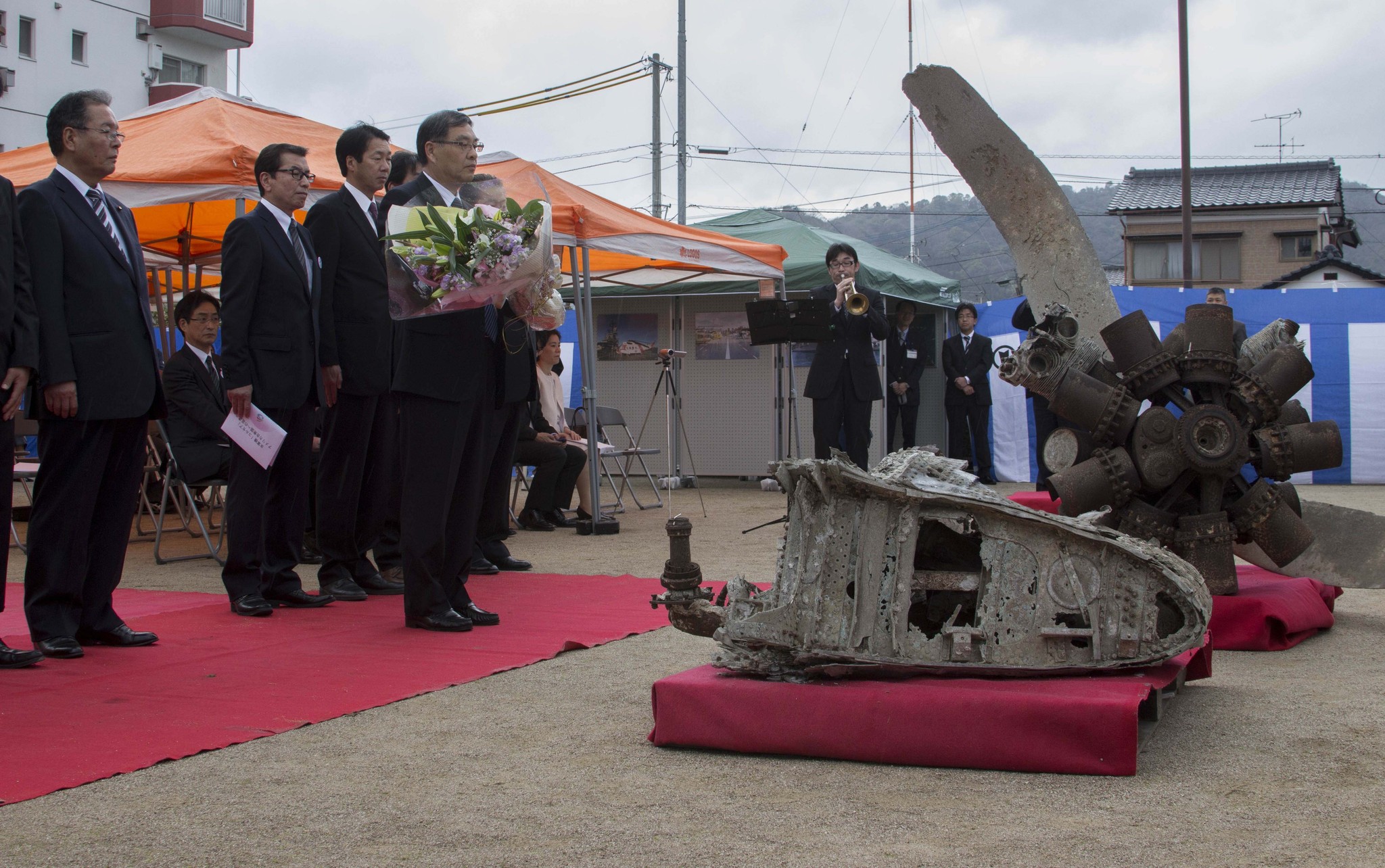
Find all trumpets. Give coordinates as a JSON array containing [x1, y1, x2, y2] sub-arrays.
[[841, 274, 869, 315]]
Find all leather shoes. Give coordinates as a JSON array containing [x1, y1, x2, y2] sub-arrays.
[[980, 476, 997, 485], [404, 606, 473, 631], [83, 624, 159, 647], [298, 546, 322, 564], [454, 601, 500, 625], [516, 507, 555, 531], [230, 567, 406, 616], [495, 557, 533, 571], [468, 559, 499, 575], [39, 634, 85, 658], [542, 507, 577, 527], [0, 639, 45, 666]]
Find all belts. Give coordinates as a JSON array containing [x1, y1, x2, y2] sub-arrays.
[[844, 353, 849, 359]]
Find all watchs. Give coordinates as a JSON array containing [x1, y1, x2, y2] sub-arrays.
[[905, 382, 910, 388]]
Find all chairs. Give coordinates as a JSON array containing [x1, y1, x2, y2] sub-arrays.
[[11, 414, 229, 569], [509, 407, 664, 530]]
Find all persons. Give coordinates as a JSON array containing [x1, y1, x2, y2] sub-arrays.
[[1205, 287, 1248, 357], [886, 300, 926, 455], [942, 303, 997, 485], [0, 87, 593, 669], [804, 242, 890, 472]]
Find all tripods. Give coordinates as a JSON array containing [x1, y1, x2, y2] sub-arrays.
[[611, 362, 707, 519], [740, 298, 832, 536]]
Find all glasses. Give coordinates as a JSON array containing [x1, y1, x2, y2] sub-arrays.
[[430, 139, 485, 152], [959, 314, 974, 319], [72, 126, 126, 143], [829, 261, 855, 269], [183, 317, 222, 324], [263, 169, 316, 183]]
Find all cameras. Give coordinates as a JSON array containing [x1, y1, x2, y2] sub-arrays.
[[659, 349, 687, 360]]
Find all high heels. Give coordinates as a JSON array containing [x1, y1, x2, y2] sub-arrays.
[[576, 505, 592, 520]]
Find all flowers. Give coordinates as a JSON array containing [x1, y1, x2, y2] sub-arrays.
[[376, 185, 548, 307]]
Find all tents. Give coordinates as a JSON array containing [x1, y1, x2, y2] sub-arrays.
[[0, 88, 963, 530]]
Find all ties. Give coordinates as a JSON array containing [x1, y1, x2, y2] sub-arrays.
[[898, 332, 905, 346], [86, 189, 130, 267], [842, 299, 849, 320], [450, 197, 499, 344], [964, 336, 970, 354], [370, 201, 378, 225], [206, 355, 224, 401]]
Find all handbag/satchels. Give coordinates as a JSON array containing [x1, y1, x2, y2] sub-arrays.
[[567, 406, 604, 443]]
